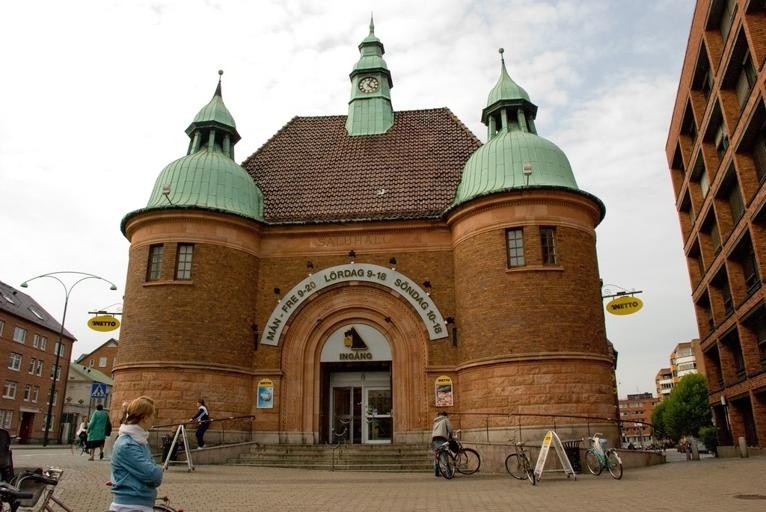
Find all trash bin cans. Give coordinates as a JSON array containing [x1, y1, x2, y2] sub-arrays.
[[561, 440, 583, 474]]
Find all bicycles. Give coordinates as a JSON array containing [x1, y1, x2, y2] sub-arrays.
[[435, 428, 481, 480], [71, 436, 94, 456], [1, 468, 182, 512], [582, 431, 624, 481], [505, 437, 538, 485]]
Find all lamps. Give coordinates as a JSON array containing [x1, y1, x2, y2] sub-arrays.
[[163, 185, 176, 205], [521, 166, 532, 184], [274, 289, 284, 299], [443, 315, 454, 325], [252, 323, 262, 334], [387, 257, 398, 269], [306, 260, 314, 272], [346, 249, 357, 262], [423, 280, 433, 293]]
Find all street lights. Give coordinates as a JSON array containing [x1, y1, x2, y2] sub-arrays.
[[19, 269, 120, 448]]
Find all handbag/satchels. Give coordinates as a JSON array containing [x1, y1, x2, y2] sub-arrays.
[[106, 418, 112, 436]]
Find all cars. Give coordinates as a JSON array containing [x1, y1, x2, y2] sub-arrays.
[[624, 439, 709, 456]]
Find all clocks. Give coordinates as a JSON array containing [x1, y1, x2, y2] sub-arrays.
[[358, 76, 378, 93]]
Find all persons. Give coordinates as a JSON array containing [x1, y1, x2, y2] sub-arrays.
[[431, 411, 455, 479], [109, 396, 163, 512], [86, 404, 110, 461], [189, 399, 210, 450], [105, 402, 129, 486], [166, 426, 177, 466], [76, 416, 90, 447]]
